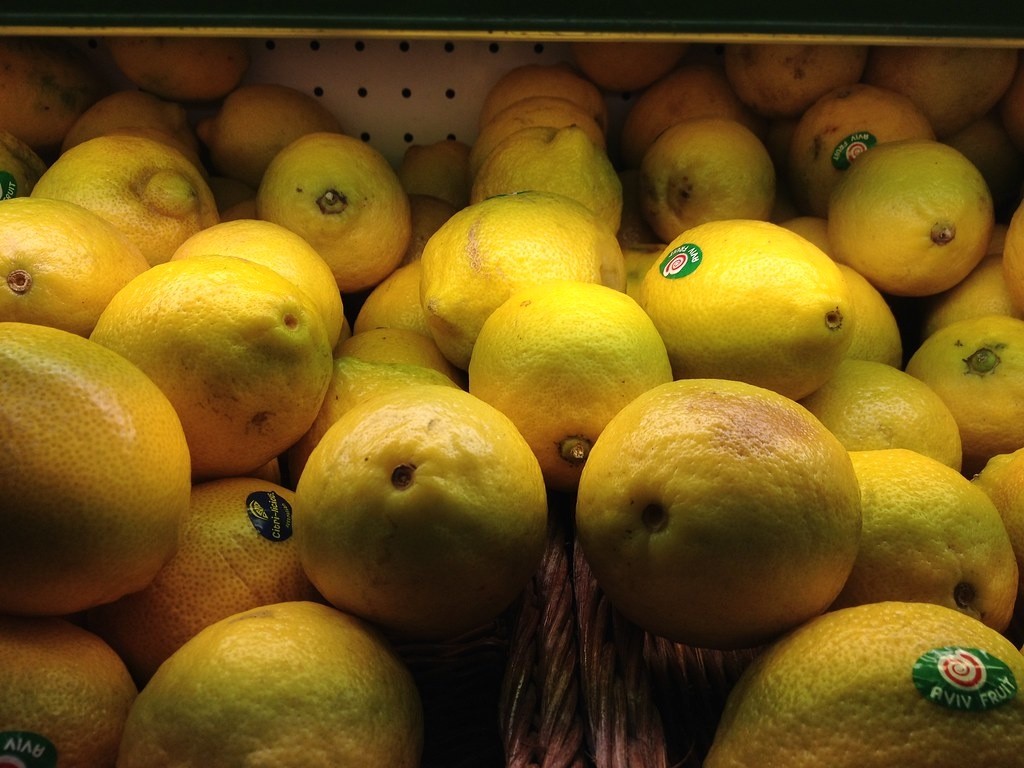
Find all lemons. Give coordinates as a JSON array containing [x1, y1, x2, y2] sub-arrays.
[[0, 33, 1024, 768]]
